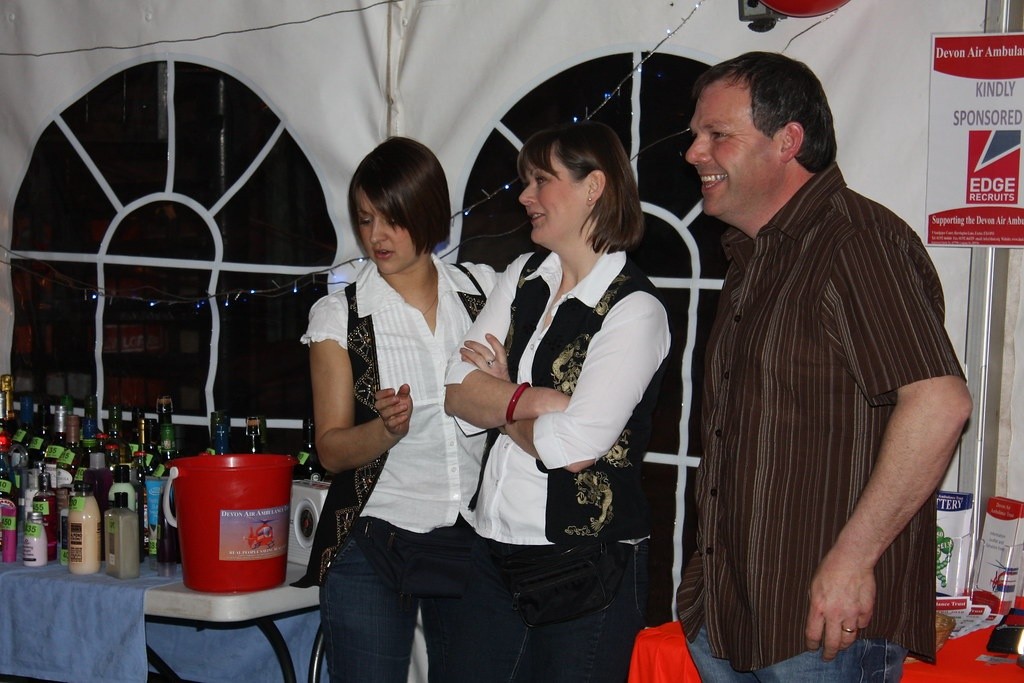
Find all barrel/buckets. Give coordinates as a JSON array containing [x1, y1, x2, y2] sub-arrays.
[[162, 453, 299, 595]]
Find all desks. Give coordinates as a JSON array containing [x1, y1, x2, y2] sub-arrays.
[[0, 554, 331, 683], [626, 590, 1023, 683]]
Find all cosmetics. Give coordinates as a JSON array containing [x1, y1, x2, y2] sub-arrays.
[[0, 453, 177, 578]]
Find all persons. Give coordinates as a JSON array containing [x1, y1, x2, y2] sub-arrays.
[[678, 50, 973, 683], [299, 137, 496, 683], [444, 121, 675, 683]]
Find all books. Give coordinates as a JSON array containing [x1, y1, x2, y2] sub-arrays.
[[936, 491, 1024, 616]]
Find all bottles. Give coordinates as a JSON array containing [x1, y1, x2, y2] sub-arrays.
[[0, 373, 181, 579], [207, 409, 326, 482]]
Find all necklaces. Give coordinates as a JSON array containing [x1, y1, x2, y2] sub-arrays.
[[423, 293, 437, 316]]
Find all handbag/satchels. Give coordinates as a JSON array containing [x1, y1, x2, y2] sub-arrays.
[[499, 537, 619, 629]]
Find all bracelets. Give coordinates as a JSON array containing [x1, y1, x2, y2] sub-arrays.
[[503, 381, 531, 425]]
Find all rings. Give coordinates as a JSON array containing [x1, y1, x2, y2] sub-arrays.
[[842, 627, 856, 632], [487, 359, 496, 367]]
